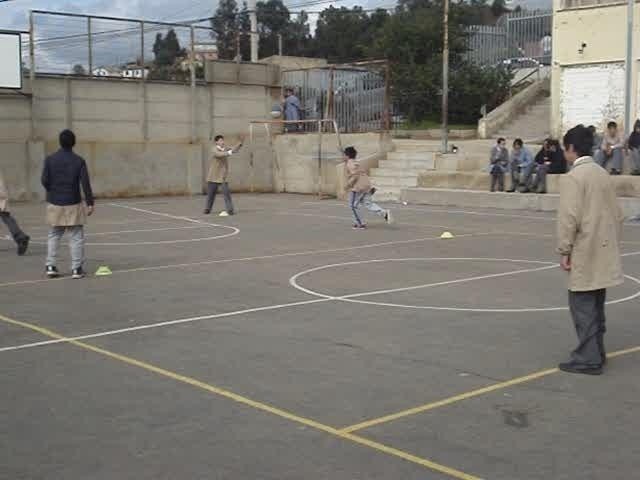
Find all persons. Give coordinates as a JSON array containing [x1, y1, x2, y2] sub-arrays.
[[343, 146, 390, 230], [517, 42, 526, 58], [168, 61, 177, 82], [489, 120, 640, 192], [0, 172, 30, 256], [539, 30, 552, 65], [284, 88, 305, 132], [555, 125, 624, 376], [41, 129, 93, 280], [203, 135, 242, 216]]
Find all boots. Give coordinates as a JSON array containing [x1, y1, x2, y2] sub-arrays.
[[1, 211, 31, 256]]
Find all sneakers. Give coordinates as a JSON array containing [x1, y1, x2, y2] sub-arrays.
[[70, 265, 88, 279], [382, 209, 390, 224], [202, 208, 213, 215], [608, 166, 639, 178], [490, 185, 547, 194], [351, 221, 368, 231], [44, 264, 64, 279], [226, 209, 237, 216], [558, 352, 608, 376]]
